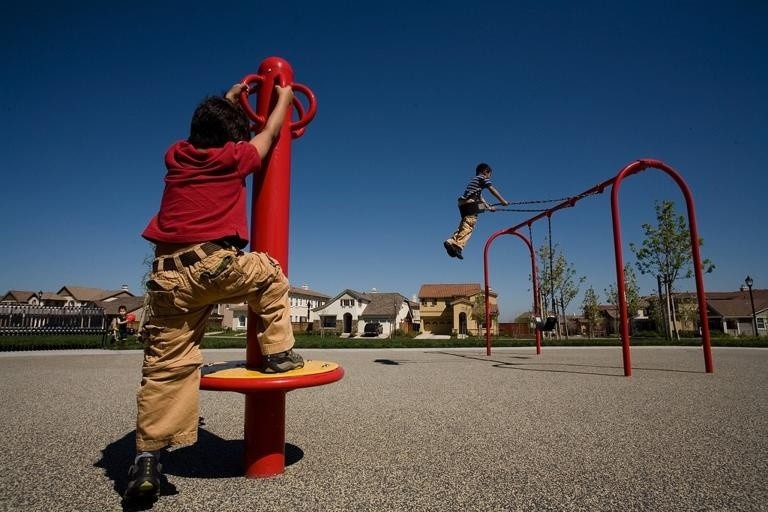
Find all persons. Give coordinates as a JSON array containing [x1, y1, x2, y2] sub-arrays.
[[121, 78, 307, 511], [443, 162, 508, 260], [115, 306, 136, 338]]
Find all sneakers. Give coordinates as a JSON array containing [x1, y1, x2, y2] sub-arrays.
[[444, 241, 463, 260], [262, 349, 304, 373], [122, 454, 162, 507]]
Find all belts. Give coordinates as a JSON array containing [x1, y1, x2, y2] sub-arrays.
[[152, 240, 228, 272]]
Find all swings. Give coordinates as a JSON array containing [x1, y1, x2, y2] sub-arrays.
[[459, 188, 602, 217]]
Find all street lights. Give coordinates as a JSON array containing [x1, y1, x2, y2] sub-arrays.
[[37, 291, 43, 306], [306, 300, 312, 322], [657, 274, 673, 340], [744, 275, 760, 337]]
